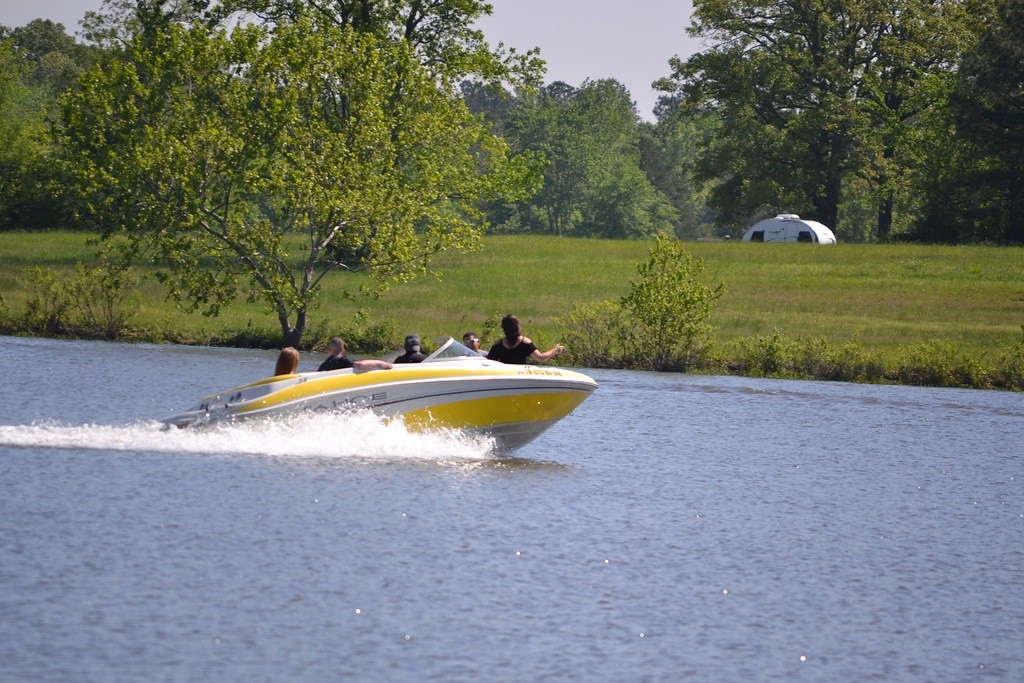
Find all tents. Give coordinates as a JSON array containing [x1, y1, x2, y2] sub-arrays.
[[739, 213, 837, 247]]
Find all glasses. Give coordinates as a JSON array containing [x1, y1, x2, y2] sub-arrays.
[[463, 338, 479, 346]]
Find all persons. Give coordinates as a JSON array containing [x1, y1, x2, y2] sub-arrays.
[[274, 346, 299, 377], [462, 332, 491, 358], [393, 334, 428, 364], [316, 336, 393, 373], [486, 313, 566, 365]]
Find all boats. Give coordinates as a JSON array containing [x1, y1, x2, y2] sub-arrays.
[[155, 336, 597, 457]]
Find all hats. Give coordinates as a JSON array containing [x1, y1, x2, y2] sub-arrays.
[[404, 334, 421, 353]]
